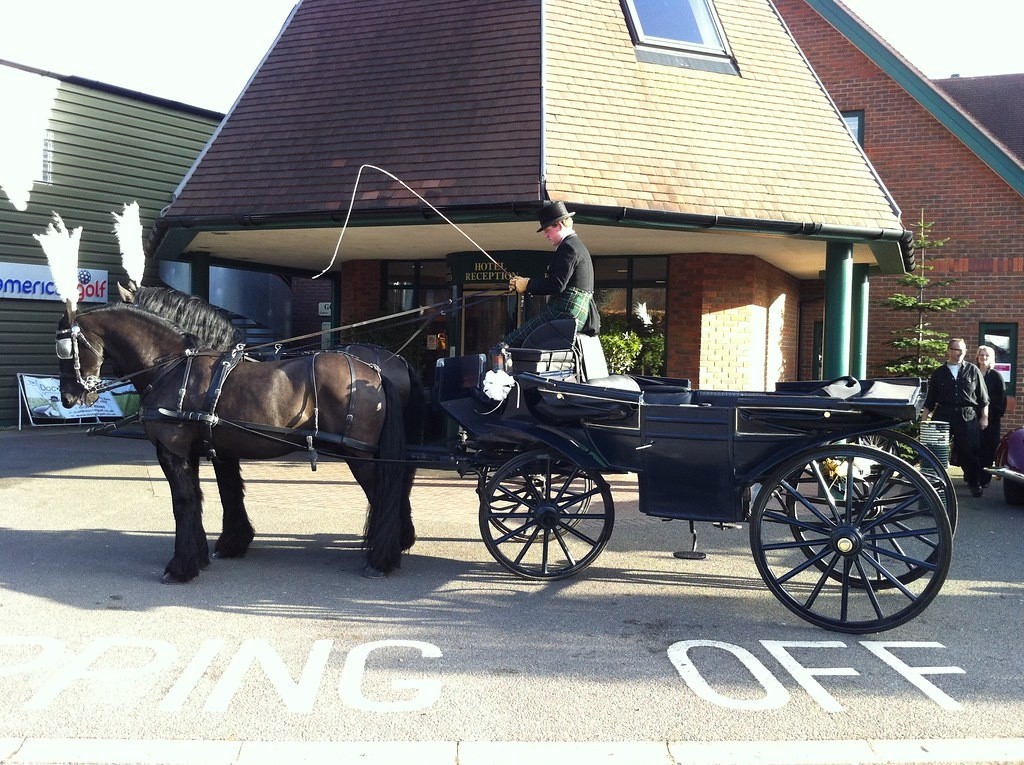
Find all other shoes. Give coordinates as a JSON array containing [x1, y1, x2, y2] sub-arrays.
[[970, 481, 984, 496]]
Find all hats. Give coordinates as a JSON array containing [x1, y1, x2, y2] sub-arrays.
[[536, 199, 575, 233]]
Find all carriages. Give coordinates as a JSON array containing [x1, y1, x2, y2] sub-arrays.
[[53, 280, 959, 636]]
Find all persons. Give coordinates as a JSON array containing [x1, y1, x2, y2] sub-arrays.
[[975, 345, 1007, 488], [489, 201, 595, 371], [922, 339, 990, 498]]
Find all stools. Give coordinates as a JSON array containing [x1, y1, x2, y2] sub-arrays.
[[529, 321, 607, 379]]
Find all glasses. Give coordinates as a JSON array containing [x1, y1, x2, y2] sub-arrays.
[[950, 348, 967, 352]]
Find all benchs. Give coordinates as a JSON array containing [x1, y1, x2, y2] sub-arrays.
[[582, 376, 691, 409], [811, 375, 862, 398]]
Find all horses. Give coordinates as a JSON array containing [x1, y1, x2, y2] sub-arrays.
[[54, 279, 424, 587]]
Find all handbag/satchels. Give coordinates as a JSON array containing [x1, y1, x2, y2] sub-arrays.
[[585, 298, 602, 336]]
